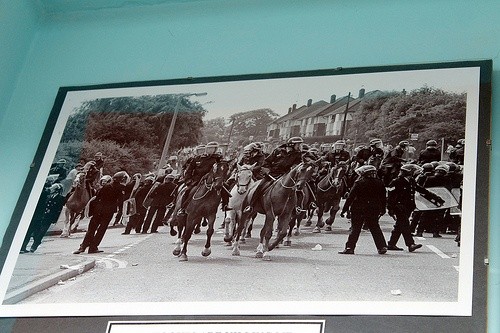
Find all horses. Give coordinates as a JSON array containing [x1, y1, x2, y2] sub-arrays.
[[61, 153, 352, 262]]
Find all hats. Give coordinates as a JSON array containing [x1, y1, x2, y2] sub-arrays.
[[165, 174, 174, 178]]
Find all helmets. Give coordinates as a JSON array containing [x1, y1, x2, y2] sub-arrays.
[[57, 159, 66, 163], [426, 140, 437, 146], [456, 140, 464, 147], [94, 152, 102, 156], [113, 171, 125, 182], [359, 165, 377, 178], [166, 137, 411, 161], [399, 161, 460, 177]]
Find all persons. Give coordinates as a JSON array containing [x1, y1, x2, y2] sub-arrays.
[[160, 141, 233, 217], [224, 136, 352, 214], [20, 152, 104, 254], [340, 139, 465, 247], [338, 166, 389, 255], [73, 168, 176, 255], [386, 165, 422, 252]]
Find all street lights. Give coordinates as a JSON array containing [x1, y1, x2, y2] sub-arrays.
[[155, 92, 208, 174]]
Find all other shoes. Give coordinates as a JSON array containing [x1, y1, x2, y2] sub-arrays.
[[415, 233, 422, 236], [387, 245, 403, 250], [409, 244, 422, 252], [22, 250, 30, 253], [89, 249, 104, 253], [73, 250, 82, 254], [246, 207, 251, 211], [151, 230, 158, 233], [122, 232, 129, 234], [178, 208, 186, 216], [433, 235, 442, 237], [30, 249, 34, 252], [378, 247, 388, 254], [222, 206, 232, 211], [338, 249, 354, 254]]
